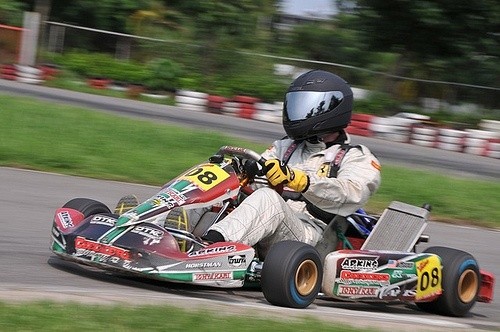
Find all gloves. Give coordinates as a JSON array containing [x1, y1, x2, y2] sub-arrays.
[[265, 157, 311, 194], [222, 157, 232, 166]]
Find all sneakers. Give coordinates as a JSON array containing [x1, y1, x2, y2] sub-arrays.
[[164, 207, 194, 253], [115, 195, 138, 219]]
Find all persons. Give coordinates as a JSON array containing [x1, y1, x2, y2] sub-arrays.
[[113, 69, 383, 261]]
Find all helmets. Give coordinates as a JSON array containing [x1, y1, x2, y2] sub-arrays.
[[282, 69, 353, 140]]
